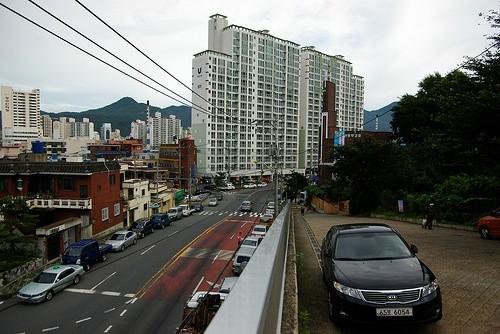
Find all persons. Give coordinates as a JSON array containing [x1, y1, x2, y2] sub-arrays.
[[301, 205, 306, 216], [421, 203, 435, 229]]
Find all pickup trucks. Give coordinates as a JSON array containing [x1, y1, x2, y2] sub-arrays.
[[59, 238, 113, 272], [178, 204, 195, 217], [218, 182, 236, 192]]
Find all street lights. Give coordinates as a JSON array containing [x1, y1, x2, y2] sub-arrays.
[[252, 160, 274, 172]]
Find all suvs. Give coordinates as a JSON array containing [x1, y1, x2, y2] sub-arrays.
[[265, 208, 275, 214], [127, 217, 155, 239], [231, 247, 256, 276]]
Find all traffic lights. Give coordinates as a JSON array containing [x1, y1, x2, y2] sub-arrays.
[[202, 176, 210, 183]]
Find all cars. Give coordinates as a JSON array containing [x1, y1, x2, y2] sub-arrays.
[[222, 179, 229, 183], [191, 203, 204, 213], [15, 263, 85, 304], [208, 198, 219, 207], [151, 212, 171, 229], [242, 183, 257, 189], [215, 194, 223, 200], [257, 182, 267, 188], [318, 221, 444, 334], [263, 213, 273, 219], [475, 205, 500, 240], [240, 235, 263, 249], [251, 178, 259, 182], [251, 225, 269, 236], [219, 275, 239, 294], [104, 230, 138, 253]]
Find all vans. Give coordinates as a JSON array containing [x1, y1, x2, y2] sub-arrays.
[[241, 178, 255, 184], [241, 200, 256, 212], [167, 206, 184, 221], [268, 201, 275, 208], [259, 217, 271, 226], [183, 290, 229, 309]]
